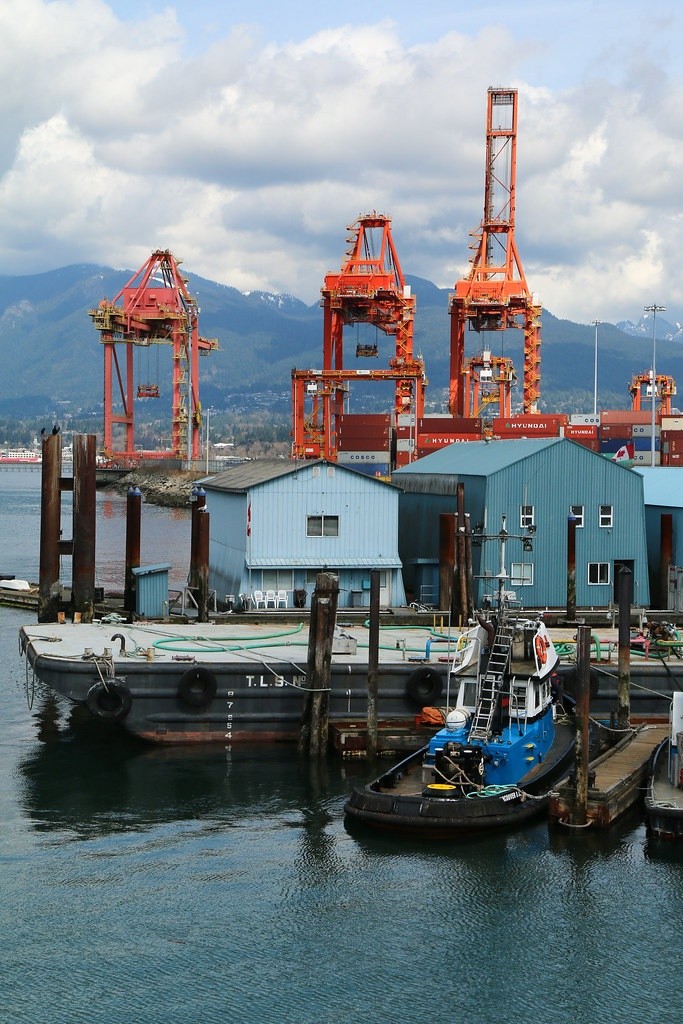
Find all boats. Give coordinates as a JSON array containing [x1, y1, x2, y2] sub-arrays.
[[342, 513, 582, 834], [0, 451, 42, 464], [646, 734, 683, 849], [19, 615, 683, 743]]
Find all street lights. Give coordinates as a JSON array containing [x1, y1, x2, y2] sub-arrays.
[[644, 304, 668, 466], [591, 318, 602, 414], [207, 406, 214, 473]]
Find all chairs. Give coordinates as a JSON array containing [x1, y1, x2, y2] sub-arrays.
[[239, 593, 255, 606], [266, 590, 276, 609], [277, 590, 288, 609], [253, 591, 266, 609]]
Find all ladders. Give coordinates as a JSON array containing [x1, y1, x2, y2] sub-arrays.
[[468, 593, 524, 746]]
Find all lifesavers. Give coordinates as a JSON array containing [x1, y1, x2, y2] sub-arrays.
[[565, 668, 600, 700], [407, 666, 444, 704], [179, 666, 218, 706], [536, 635, 547, 664], [85, 679, 133, 723]]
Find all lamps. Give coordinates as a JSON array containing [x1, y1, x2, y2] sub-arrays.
[[524, 541, 533, 551], [528, 524, 536, 533], [475, 521, 484, 531], [472, 538, 481, 547]]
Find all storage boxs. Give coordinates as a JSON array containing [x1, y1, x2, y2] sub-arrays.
[[336, 410, 683, 477]]
